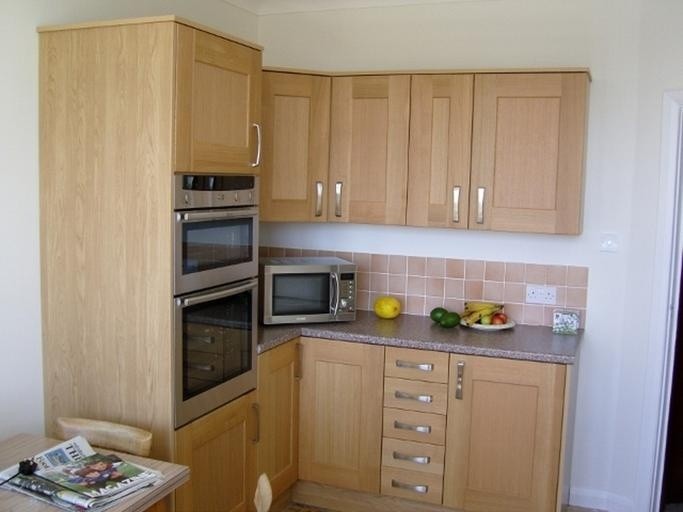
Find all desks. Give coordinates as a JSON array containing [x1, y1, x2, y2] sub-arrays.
[[0, 430, 191, 512]]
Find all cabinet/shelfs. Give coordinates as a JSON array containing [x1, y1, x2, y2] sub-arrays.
[[260, 71, 411, 226], [298, 328, 385, 511], [37, 16, 260, 174], [407, 72, 591, 237], [380, 337, 450, 512], [257, 333, 298, 512], [172, 387, 257, 512], [443, 344, 568, 512]]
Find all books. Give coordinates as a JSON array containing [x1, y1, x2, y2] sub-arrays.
[[33, 452, 156, 498]]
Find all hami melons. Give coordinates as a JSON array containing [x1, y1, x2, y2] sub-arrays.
[[373, 295, 401, 320]]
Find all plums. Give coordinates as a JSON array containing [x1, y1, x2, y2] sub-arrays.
[[481, 315, 492, 324]]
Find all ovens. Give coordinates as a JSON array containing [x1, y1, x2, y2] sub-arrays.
[[171, 174, 260, 430]]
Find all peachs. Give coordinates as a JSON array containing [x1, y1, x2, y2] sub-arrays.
[[495, 313, 507, 323], [493, 316, 502, 324]]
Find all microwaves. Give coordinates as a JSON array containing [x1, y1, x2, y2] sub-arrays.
[[259, 256, 357, 326]]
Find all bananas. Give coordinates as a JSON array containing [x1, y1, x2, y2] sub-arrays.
[[462, 302, 504, 327]]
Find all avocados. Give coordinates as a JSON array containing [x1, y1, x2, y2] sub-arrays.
[[430, 308, 448, 321], [440, 312, 460, 328]]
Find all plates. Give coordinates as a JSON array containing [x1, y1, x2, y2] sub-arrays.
[[460, 320, 516, 332]]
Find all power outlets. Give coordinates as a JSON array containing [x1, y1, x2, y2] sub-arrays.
[[525, 286, 557, 305]]
